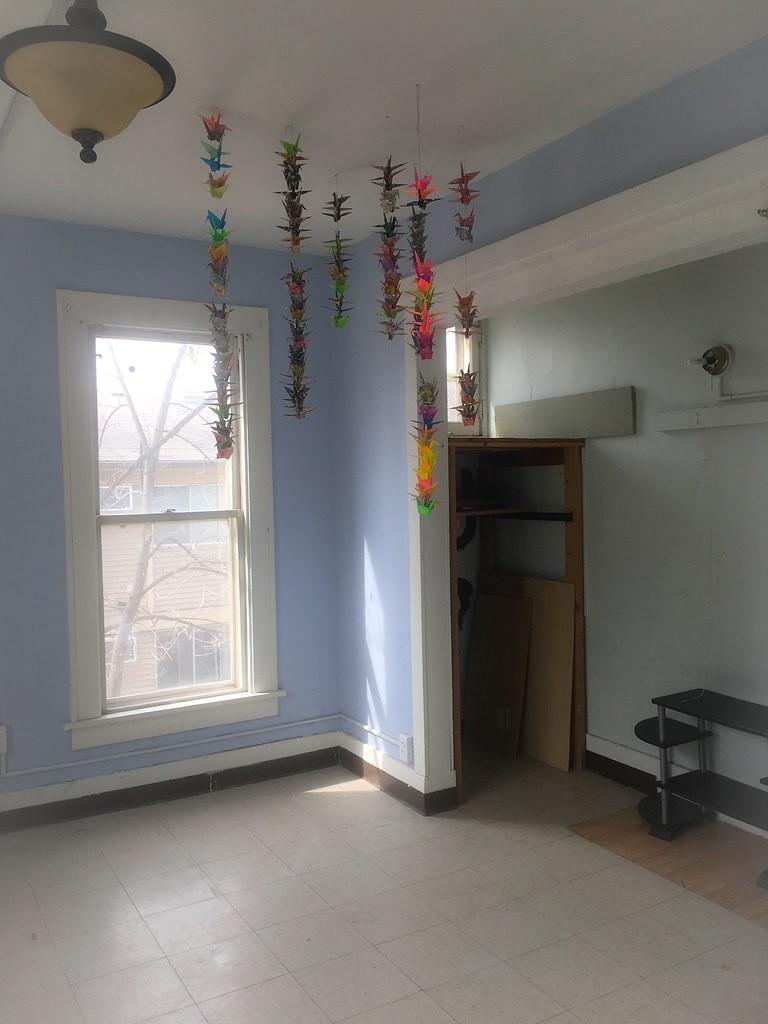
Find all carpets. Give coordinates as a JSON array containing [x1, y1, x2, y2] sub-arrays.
[[566, 805, 768, 928]]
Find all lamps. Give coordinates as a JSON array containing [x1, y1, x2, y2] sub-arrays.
[[685, 344, 734, 377], [0, 0, 177, 164]]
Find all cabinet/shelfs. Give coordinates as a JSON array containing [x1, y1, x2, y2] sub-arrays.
[[449, 438, 587, 804], [633, 687, 768, 888]]
